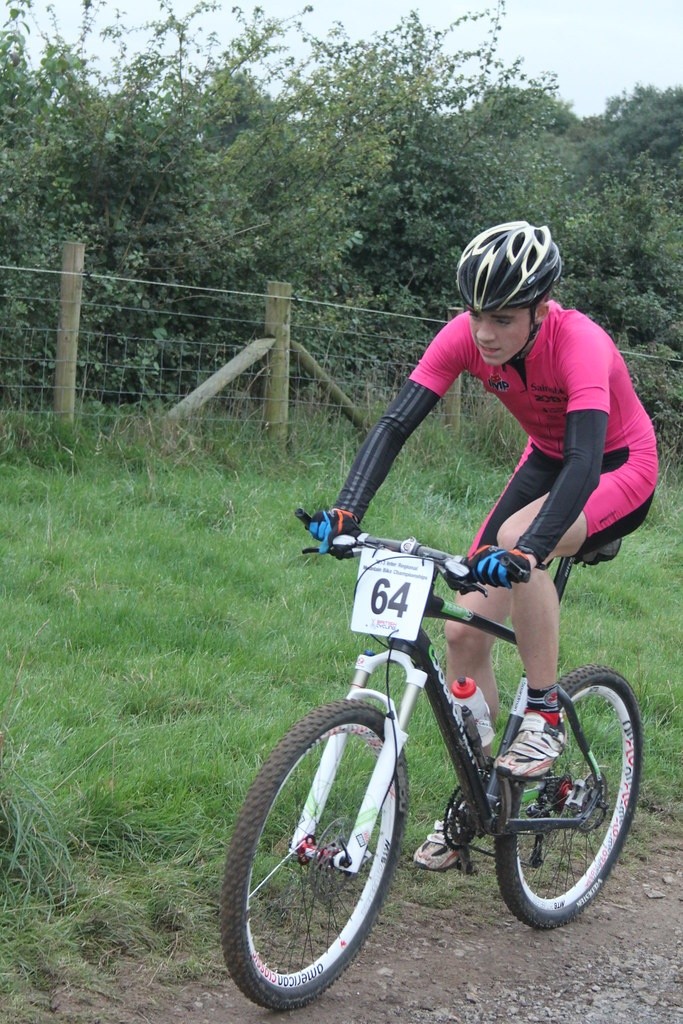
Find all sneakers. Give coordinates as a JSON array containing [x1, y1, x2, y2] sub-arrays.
[[494, 712, 567, 780], [413, 799, 470, 871]]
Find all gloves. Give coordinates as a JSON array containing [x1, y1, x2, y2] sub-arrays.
[[310, 507, 360, 553], [470, 544, 530, 589]]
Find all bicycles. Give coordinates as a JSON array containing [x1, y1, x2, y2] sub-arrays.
[[219, 505, 644, 1012]]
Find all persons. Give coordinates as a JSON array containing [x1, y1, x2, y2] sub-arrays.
[[305, 222, 658, 873]]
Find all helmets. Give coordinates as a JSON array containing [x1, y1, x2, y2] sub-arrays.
[[456, 219, 563, 311]]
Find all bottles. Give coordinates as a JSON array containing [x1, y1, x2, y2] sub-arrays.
[[453, 676, 494, 747]]
